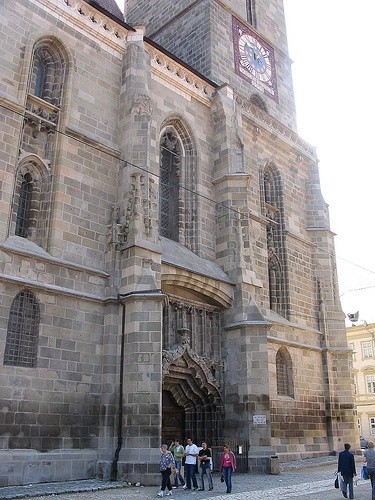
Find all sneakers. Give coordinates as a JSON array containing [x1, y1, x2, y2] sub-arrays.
[[165, 490, 173, 496], [157, 490, 163, 497]]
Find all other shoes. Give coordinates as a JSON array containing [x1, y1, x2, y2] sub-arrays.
[[184, 488, 190, 490], [172, 487, 178, 488], [194, 486, 198, 490], [342, 491, 348, 498], [208, 487, 214, 491], [198, 488, 205, 491], [227, 490, 231, 493], [183, 484, 186, 488]]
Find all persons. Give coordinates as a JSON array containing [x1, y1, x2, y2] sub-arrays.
[[220, 445, 237, 493], [363, 441, 375, 500], [167, 439, 186, 489], [338, 443, 357, 500], [183, 438, 200, 491], [156, 444, 176, 497], [198, 442, 213, 491]]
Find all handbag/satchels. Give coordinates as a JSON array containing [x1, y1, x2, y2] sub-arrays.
[[335, 474, 339, 489], [220, 470, 224, 482], [361, 460, 370, 480], [170, 464, 179, 478]]
